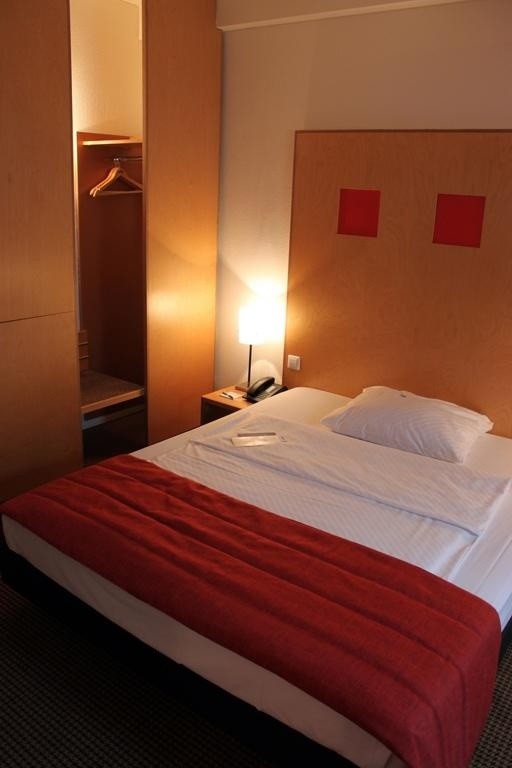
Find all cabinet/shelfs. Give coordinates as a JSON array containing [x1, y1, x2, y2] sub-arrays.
[[1, 0, 223, 498]]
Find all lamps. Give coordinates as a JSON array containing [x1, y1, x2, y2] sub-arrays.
[[235, 299, 267, 393]]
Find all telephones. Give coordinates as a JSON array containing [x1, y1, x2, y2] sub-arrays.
[[242, 376, 288, 403]]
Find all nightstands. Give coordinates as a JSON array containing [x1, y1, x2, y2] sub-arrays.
[[200, 400, 241, 427]]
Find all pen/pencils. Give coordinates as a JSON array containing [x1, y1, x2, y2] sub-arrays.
[[222, 392, 234, 399]]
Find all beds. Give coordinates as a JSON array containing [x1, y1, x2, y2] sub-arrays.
[[3, 386, 510, 765]]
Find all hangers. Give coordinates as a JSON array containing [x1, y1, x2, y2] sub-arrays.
[[87, 156, 143, 198]]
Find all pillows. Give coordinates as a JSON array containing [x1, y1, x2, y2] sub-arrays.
[[317, 385, 497, 464]]
